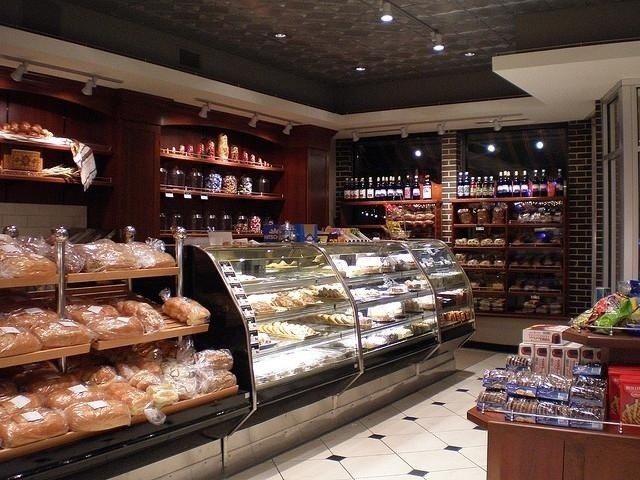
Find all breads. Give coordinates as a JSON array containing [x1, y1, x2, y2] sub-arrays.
[[0, 324, 41, 359], [353, 277, 430, 298], [0, 407, 70, 448], [1, 380, 17, 398], [511, 254, 564, 269], [262, 323, 316, 339], [334, 253, 419, 276], [493, 279, 505, 291], [50, 383, 117, 408], [405, 295, 441, 315], [369, 299, 403, 323], [0, 393, 48, 418], [275, 290, 315, 310], [30, 318, 89, 349], [397, 213, 435, 228], [470, 278, 484, 287], [477, 207, 491, 225], [7, 306, 56, 329], [425, 259, 464, 288], [411, 319, 438, 336], [468, 238, 480, 248], [512, 279, 561, 292], [309, 286, 346, 299], [440, 290, 473, 324], [480, 238, 492, 248], [456, 255, 504, 267], [305, 314, 373, 328], [22, 372, 81, 394], [0, 233, 83, 281], [459, 207, 474, 224], [103, 341, 237, 417], [455, 237, 467, 246], [75, 364, 115, 387], [117, 300, 162, 334], [64, 400, 132, 432], [473, 298, 506, 314], [493, 207, 504, 224], [87, 318, 144, 342], [2, 123, 50, 142], [163, 297, 208, 325], [80, 239, 174, 273], [389, 328, 414, 340], [364, 332, 388, 349], [249, 301, 273, 316], [493, 237, 506, 246], [521, 297, 563, 314], [71, 304, 118, 323]]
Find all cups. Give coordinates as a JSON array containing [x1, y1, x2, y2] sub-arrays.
[[208, 231, 234, 248]]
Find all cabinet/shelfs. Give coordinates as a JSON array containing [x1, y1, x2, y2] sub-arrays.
[[1, 225, 252, 478], [161, 146, 288, 237], [339, 199, 442, 244], [466, 327, 640, 480], [448, 196, 570, 320], [0, 131, 115, 192]]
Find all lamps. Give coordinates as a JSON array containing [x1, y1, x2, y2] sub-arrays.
[[437, 124, 444, 135], [80, 77, 98, 97], [9, 61, 32, 84], [282, 121, 292, 134], [351, 130, 363, 142], [198, 102, 211, 118], [378, 1, 393, 23], [492, 119, 501, 131], [429, 30, 445, 51], [247, 112, 264, 127], [400, 126, 410, 137]]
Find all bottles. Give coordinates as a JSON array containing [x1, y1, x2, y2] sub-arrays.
[[222, 173, 238, 194], [532, 170, 540, 196], [343, 174, 402, 200], [238, 178, 253, 196], [457, 172, 496, 199], [187, 170, 202, 191], [556, 169, 564, 197], [159, 168, 169, 196], [540, 170, 547, 196], [256, 177, 270, 195], [513, 171, 520, 197], [189, 213, 203, 233], [412, 175, 422, 199], [172, 169, 185, 188], [404, 176, 411, 201], [171, 213, 183, 231], [205, 213, 218, 230], [422, 175, 432, 200], [236, 214, 247, 231], [159, 213, 169, 233], [521, 171, 530, 196], [498, 170, 512, 198], [220, 211, 232, 230], [204, 174, 222, 194]]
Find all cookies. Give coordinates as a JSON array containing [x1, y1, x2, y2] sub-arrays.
[[479, 355, 607, 431]]
